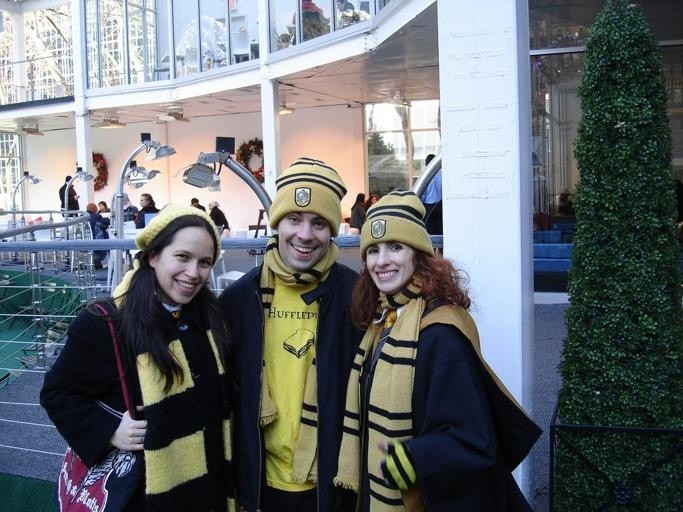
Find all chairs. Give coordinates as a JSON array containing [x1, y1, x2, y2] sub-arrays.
[[150, 8, 363, 82], [0, 300, 81, 392], [0, 212, 263, 300], [533, 216, 573, 274]]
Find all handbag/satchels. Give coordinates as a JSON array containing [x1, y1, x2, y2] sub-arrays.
[[54, 299, 145, 512]]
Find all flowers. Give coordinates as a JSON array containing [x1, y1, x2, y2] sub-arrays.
[[92, 152, 109, 192], [234, 137, 264, 184]]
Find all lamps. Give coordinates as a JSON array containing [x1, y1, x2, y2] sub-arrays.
[[62, 167, 93, 267], [109, 160, 160, 265], [278, 102, 295, 117], [157, 111, 191, 123], [182, 149, 273, 219], [11, 119, 43, 137], [111, 132, 179, 283], [89, 116, 127, 129], [11, 171, 42, 264]]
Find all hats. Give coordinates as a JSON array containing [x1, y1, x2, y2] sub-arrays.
[[266, 156, 348, 238], [132, 201, 221, 269], [358, 186, 436, 258]]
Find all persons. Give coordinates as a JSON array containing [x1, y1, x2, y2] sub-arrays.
[[350, 155, 441, 233], [331, 188, 542, 510], [532, 212, 555, 232], [38, 204, 242, 512], [217, 157, 362, 510], [290, 1, 359, 47], [60, 176, 230, 268], [556, 190, 577, 216]]
